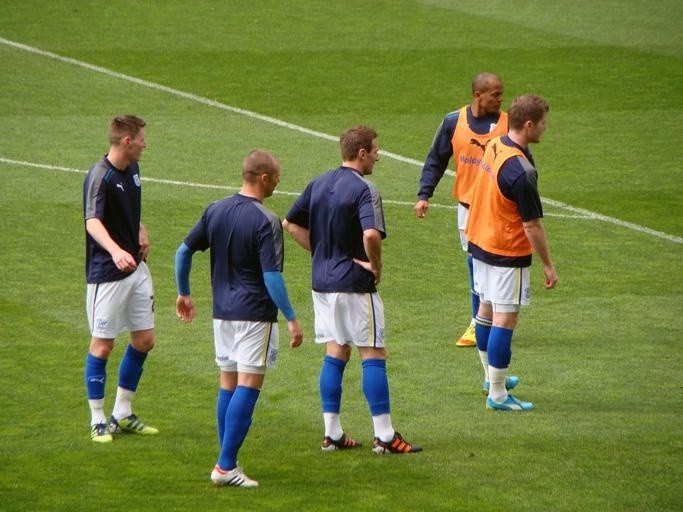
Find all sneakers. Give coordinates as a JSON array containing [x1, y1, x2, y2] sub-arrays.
[[486, 394, 534, 414], [91, 420, 113, 443], [109, 414, 160, 435], [372, 432, 423, 455], [211, 464, 259, 487], [483, 376, 519, 396], [321, 432, 362, 452], [456, 318, 477, 347]]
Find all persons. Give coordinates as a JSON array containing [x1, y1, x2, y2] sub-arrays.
[[466, 94, 559, 410], [83, 115, 159, 443], [283, 124, 422, 453], [415, 71, 509, 348], [175, 148, 303, 489]]
